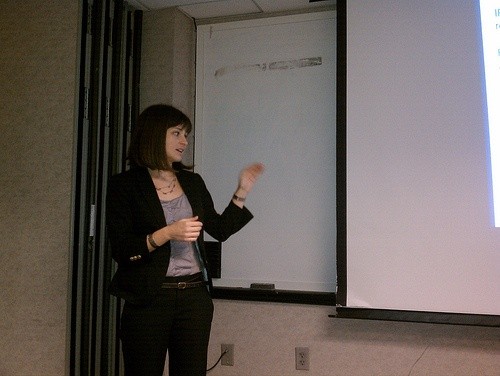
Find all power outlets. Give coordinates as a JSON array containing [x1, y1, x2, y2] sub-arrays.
[[295, 347, 309, 370]]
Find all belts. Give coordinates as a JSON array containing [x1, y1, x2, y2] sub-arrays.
[[163, 282, 211, 288]]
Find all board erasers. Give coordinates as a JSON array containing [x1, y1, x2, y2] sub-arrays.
[[250, 282, 276, 291]]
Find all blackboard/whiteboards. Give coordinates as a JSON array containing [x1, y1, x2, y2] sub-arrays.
[[189, 6, 338, 307]]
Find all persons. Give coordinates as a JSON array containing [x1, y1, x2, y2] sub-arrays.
[[105, 104, 264, 376]]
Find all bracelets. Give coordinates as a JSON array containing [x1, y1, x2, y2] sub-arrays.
[[233, 193, 245, 202], [148, 233, 160, 249]]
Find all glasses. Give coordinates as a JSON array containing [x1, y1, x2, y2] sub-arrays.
[[169, 128, 192, 142]]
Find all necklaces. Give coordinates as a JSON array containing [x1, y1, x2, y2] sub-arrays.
[[155, 173, 176, 195]]
